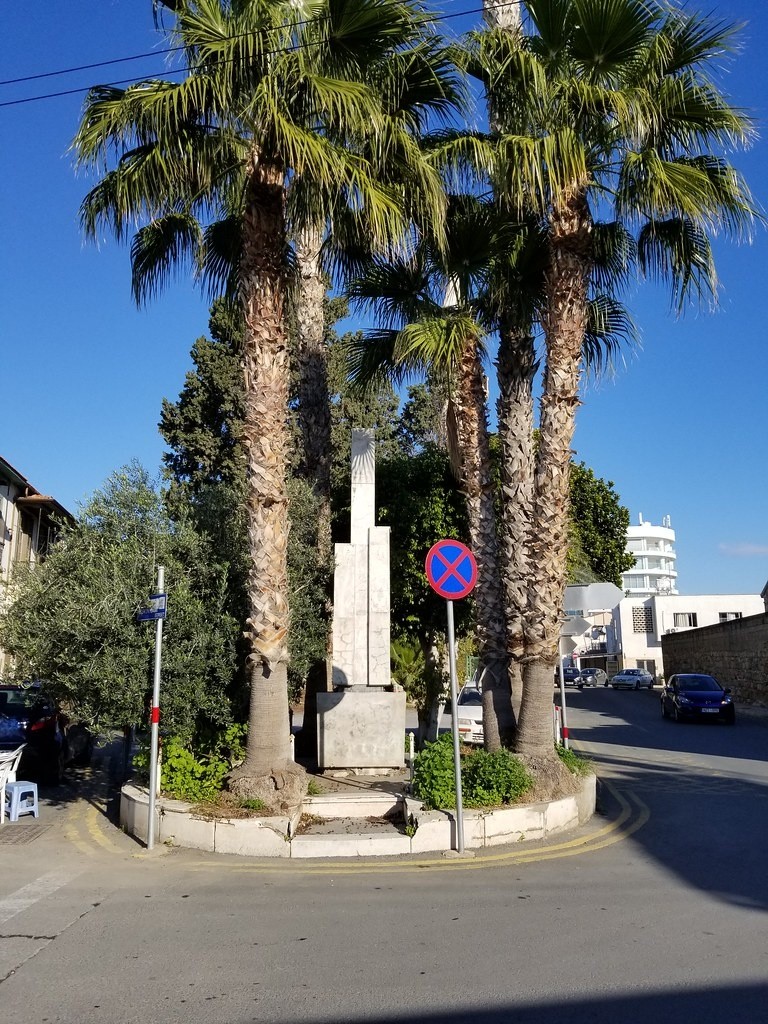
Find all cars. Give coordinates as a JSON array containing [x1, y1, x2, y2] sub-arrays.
[[556, 667, 580, 688], [453, 678, 485, 749], [0, 684, 96, 794], [573, 668, 609, 688], [611, 668, 654, 691], [660, 674, 736, 727]]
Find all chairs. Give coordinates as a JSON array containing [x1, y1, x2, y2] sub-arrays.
[[0, 742, 27, 824], [466, 692, 479, 701]]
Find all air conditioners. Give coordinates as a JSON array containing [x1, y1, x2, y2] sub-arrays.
[[668, 628, 678, 633]]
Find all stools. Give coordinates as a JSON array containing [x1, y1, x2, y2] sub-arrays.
[[5, 780, 38, 821]]
